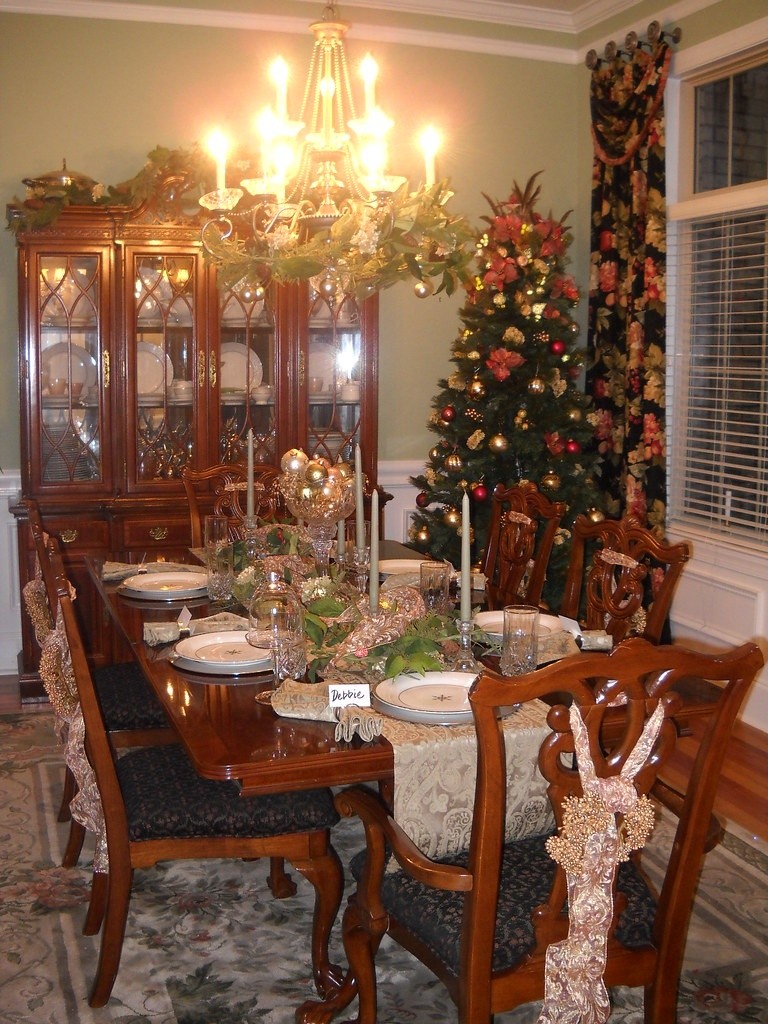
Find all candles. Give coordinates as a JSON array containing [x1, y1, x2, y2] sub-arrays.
[[354, 443, 366, 549], [248, 427, 254, 516], [337, 454, 345, 556], [460, 491, 471, 622], [370, 488, 379, 612]]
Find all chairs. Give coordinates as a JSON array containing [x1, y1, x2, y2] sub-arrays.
[[295, 638, 764, 1024], [476, 484, 567, 607], [46, 537, 346, 1008], [24, 498, 260, 869], [180, 464, 295, 548], [560, 513, 689, 646]]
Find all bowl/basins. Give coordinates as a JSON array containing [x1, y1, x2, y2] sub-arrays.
[[159, 298, 194, 320]]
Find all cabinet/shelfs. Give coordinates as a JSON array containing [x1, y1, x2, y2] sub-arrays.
[[6, 146, 394, 708]]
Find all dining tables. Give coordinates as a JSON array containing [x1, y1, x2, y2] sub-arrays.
[[80, 540, 726, 821]]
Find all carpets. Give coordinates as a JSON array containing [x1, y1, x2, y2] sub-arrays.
[[0, 707, 768, 1023]]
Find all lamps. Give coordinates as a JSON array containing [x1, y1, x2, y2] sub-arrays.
[[195, 1, 477, 301]]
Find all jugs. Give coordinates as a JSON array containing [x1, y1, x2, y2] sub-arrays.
[[246, 571, 306, 649]]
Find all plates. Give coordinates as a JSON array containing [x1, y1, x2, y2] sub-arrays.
[[115, 558, 565, 725], [39, 265, 364, 484]]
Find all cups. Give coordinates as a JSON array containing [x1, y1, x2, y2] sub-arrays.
[[309, 378, 323, 392], [66, 383, 84, 395], [207, 545, 233, 603], [47, 379, 66, 394], [204, 515, 228, 552], [345, 520, 370, 584], [420, 563, 450, 627], [336, 312, 358, 324], [267, 608, 307, 681], [500, 605, 539, 676], [340, 383, 359, 404]]
